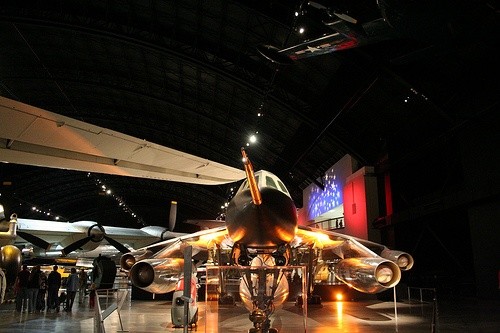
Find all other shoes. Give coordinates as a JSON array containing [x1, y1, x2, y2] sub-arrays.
[[65, 308, 71, 311], [23, 311, 26, 313], [28, 311, 32, 314], [41, 307, 45, 311]]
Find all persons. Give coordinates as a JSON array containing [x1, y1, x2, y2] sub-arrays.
[[27, 267, 40, 314], [64, 268, 79, 312], [16, 264, 31, 314], [46, 265, 61, 313], [35, 265, 47, 313]]
